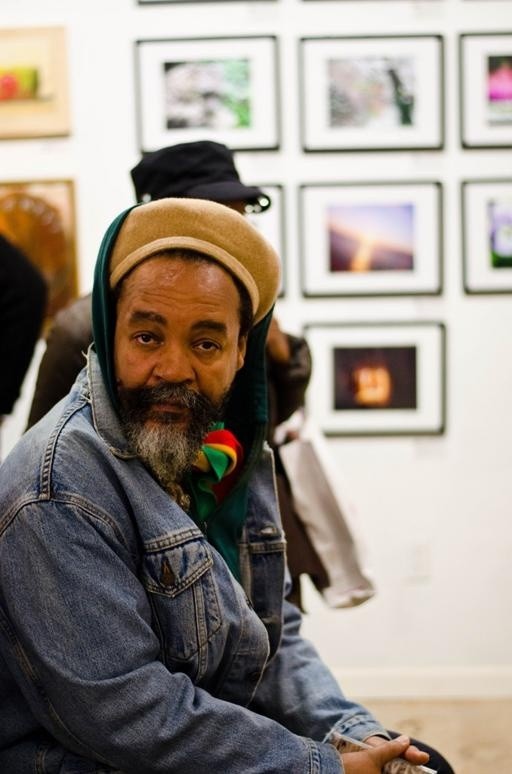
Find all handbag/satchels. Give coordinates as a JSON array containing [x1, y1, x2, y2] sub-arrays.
[[270, 411, 376, 610]]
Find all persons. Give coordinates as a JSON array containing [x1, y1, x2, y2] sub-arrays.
[[1, 198, 433, 774], [26, 139, 371, 627], [4, 232, 50, 426]]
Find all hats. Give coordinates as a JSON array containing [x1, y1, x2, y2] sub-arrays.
[[130, 139, 271, 214], [107, 195, 281, 326]]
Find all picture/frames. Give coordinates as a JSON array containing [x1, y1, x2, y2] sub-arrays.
[[240, 182, 289, 301], [297, 178, 444, 298], [0, 176, 79, 348], [460, 175, 511, 295], [296, 32, 446, 154], [131, 30, 280, 154], [457, 31, 511, 150], [300, 320, 447, 438]]
